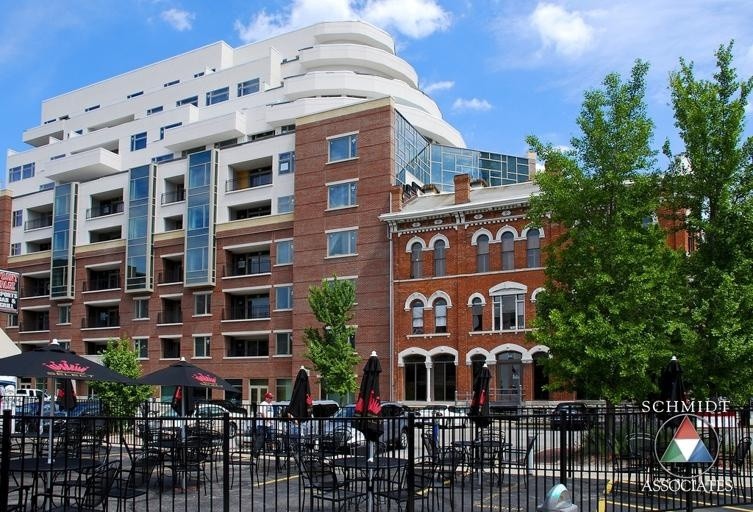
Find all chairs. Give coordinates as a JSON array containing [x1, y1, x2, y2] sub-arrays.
[[0, 414, 752, 511]]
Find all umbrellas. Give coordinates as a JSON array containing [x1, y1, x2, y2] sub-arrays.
[[285, 364, 312, 436], [656, 356, 691, 428], [0, 345, 240, 463], [351, 351, 382, 462], [467, 363, 492, 441]]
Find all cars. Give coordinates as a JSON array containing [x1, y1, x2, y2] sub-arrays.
[[41, 401, 108, 437], [414, 404, 471, 428], [133, 403, 245, 448], [319, 401, 410, 452], [549, 402, 599, 431]]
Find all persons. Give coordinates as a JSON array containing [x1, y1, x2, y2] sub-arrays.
[[279, 406, 298, 434], [250, 393, 275, 464], [685, 398, 691, 408]]
